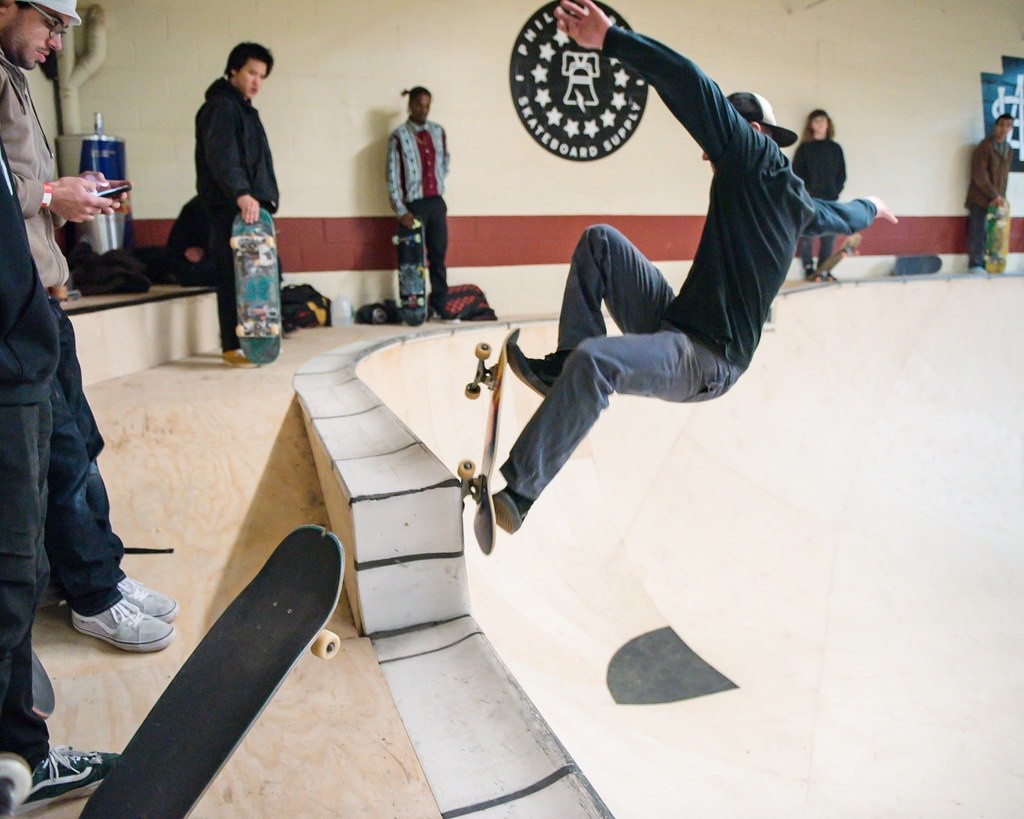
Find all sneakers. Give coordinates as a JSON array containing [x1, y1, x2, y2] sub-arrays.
[[72, 600, 174, 654], [12, 746, 121, 816], [116, 577, 180, 625]]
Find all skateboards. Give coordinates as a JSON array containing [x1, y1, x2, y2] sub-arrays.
[[229, 207, 282, 363], [458, 326, 527, 555], [0, 752, 33, 819], [983, 196, 1010, 275], [807, 233, 863, 281], [77, 524, 345, 819], [392, 216, 426, 326], [891, 255, 942, 275]]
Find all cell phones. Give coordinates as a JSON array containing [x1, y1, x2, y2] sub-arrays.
[[96, 185, 131, 196]]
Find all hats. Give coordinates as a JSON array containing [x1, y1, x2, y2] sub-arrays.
[[22, 0, 82, 27], [728, 92, 799, 148]]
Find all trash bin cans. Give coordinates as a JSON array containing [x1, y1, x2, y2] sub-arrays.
[[52, 132, 130, 258]]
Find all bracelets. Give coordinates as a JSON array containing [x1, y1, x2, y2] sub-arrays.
[[42, 183, 53, 207]]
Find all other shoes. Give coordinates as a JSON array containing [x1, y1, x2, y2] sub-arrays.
[[823, 272, 835, 281], [507, 344, 560, 397], [966, 266, 987, 276], [492, 486, 534, 533], [807, 270, 822, 281]]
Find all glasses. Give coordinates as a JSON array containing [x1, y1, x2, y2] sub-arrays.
[[27, 2, 66, 39]]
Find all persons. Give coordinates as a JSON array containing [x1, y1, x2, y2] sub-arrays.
[[195, 43, 281, 367], [167, 195, 296, 333], [0, 149, 127, 818], [492, 0, 899, 534], [385, 87, 459, 323], [792, 110, 846, 282], [0, 0, 180, 654], [963, 114, 1014, 275]]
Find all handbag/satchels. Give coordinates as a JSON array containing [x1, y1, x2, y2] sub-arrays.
[[426, 284, 497, 322], [280, 284, 331, 334]]
